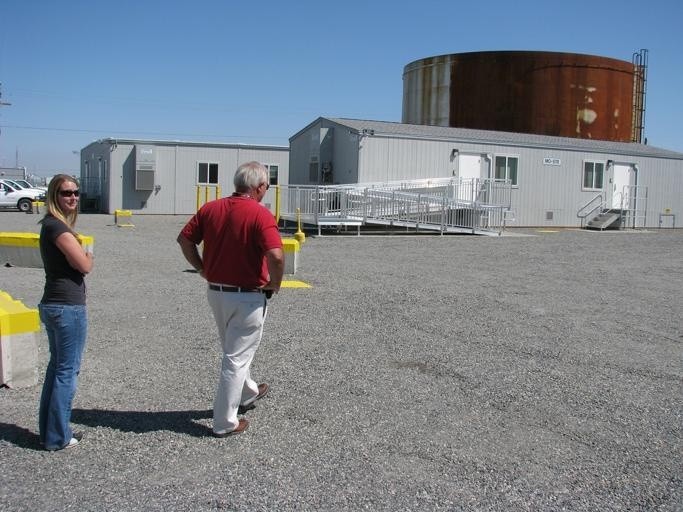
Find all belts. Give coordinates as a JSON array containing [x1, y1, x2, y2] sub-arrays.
[[209, 285, 258, 293]]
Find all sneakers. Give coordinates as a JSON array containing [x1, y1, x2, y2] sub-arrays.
[[66, 431, 84, 448]]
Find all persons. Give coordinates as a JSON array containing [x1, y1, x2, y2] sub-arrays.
[[175, 160, 286, 438], [36, 174, 94, 450]]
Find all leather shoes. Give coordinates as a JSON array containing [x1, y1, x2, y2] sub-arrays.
[[240, 383, 269, 410], [213, 419, 250, 437]]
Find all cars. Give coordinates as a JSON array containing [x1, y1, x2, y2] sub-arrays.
[[0, 179, 46, 200], [0, 182, 40, 212], [15, 180, 48, 194]]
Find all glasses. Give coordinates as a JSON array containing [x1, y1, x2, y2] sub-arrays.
[[60, 189, 80, 197], [266, 184, 269, 190]]
